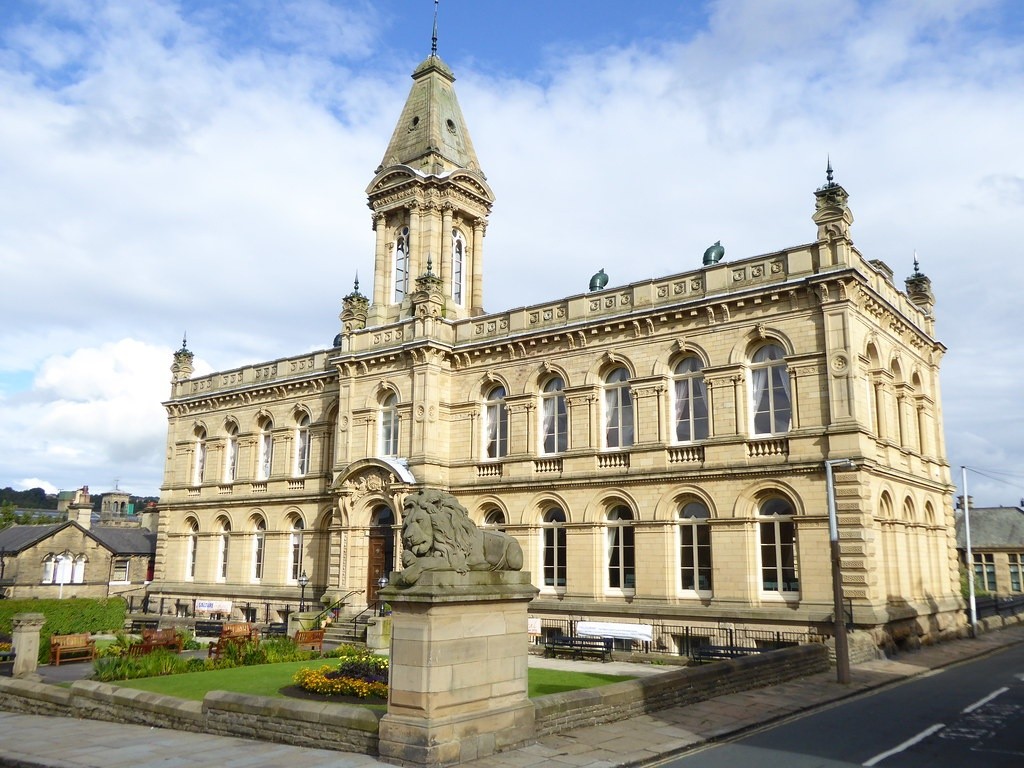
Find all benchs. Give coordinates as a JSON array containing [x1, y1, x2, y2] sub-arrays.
[[691, 645, 771, 667], [544, 635, 613, 663], [208, 623, 325, 657], [129, 618, 159, 635], [128, 626, 183, 656], [50, 631, 96, 666], [0, 647, 16, 677], [195, 621, 225, 637]]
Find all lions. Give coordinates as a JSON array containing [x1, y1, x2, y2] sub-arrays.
[[399, 488, 524, 584]]
